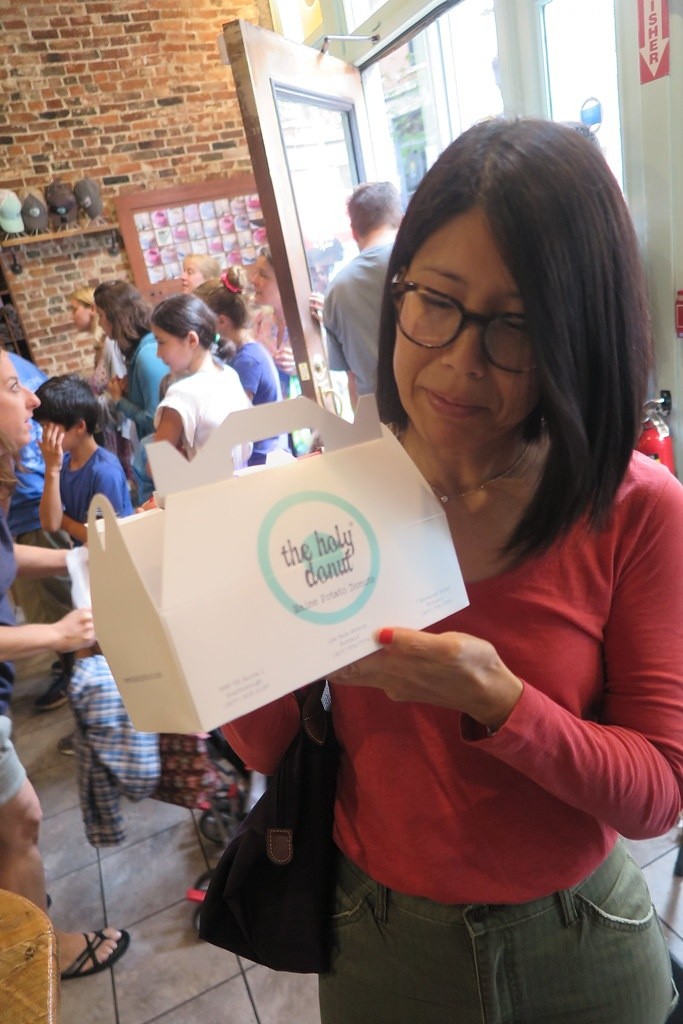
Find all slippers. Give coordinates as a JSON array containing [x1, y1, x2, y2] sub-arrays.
[[60, 929, 130, 979]]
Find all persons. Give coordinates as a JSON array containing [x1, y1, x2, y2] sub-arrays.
[[1, 177, 403, 983], [217, 115, 683, 1024]]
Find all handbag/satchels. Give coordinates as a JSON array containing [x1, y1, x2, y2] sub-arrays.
[[197, 679, 350, 975]]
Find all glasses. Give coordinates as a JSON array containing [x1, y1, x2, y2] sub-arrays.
[[389, 259, 544, 374]]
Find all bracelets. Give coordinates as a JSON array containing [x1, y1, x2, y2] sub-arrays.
[[483, 727, 497, 737]]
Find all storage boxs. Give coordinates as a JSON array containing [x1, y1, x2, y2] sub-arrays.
[[81, 391, 469, 735]]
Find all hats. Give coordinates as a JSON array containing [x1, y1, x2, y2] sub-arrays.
[[0, 196, 24, 233], [45, 181, 69, 205], [18, 186, 48, 209], [21, 193, 48, 229], [0, 186, 22, 208], [73, 179, 104, 220], [50, 187, 76, 225]]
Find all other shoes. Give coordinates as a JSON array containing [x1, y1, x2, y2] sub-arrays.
[[56, 733, 74, 754]]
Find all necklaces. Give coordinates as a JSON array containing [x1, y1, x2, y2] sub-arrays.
[[428, 440, 534, 504]]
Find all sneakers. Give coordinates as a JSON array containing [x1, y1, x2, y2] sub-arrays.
[[33, 668, 75, 710], [50, 657, 72, 674]]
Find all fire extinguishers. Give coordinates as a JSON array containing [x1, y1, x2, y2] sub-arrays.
[[635, 396, 674, 477]]
